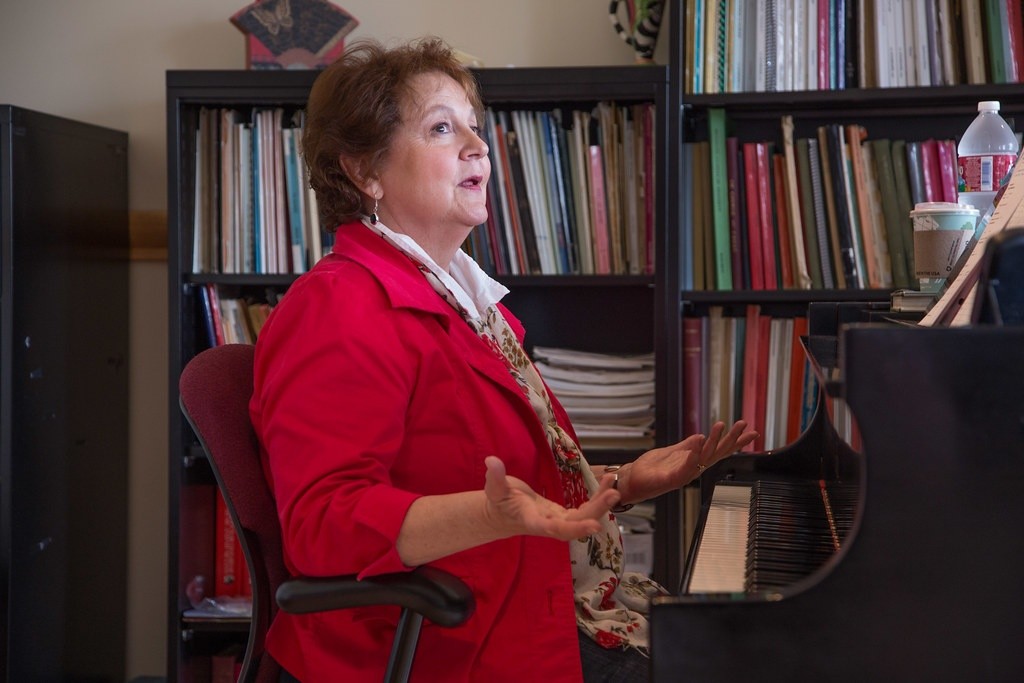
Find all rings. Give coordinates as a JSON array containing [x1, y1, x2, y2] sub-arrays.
[[696, 464, 705, 471]]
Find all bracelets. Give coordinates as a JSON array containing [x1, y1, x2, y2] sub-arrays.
[[604, 463, 633, 514]]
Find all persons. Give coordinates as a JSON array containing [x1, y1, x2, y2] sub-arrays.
[[246, 34, 760, 683]]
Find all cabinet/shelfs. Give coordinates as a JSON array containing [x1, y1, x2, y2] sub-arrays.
[[154, 2, 1024, 682]]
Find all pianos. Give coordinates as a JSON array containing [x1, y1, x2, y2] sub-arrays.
[[646, 230, 1024, 683]]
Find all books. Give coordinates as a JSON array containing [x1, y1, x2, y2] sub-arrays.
[[677, 0, 1023, 586], [191, 103, 659, 683]]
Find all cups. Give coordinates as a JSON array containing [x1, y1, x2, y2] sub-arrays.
[[909, 201, 980, 294]]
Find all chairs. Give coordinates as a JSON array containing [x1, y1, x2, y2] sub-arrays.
[[174, 343, 476, 682]]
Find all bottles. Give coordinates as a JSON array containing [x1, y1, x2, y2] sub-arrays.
[[958, 100, 1019, 233]]
